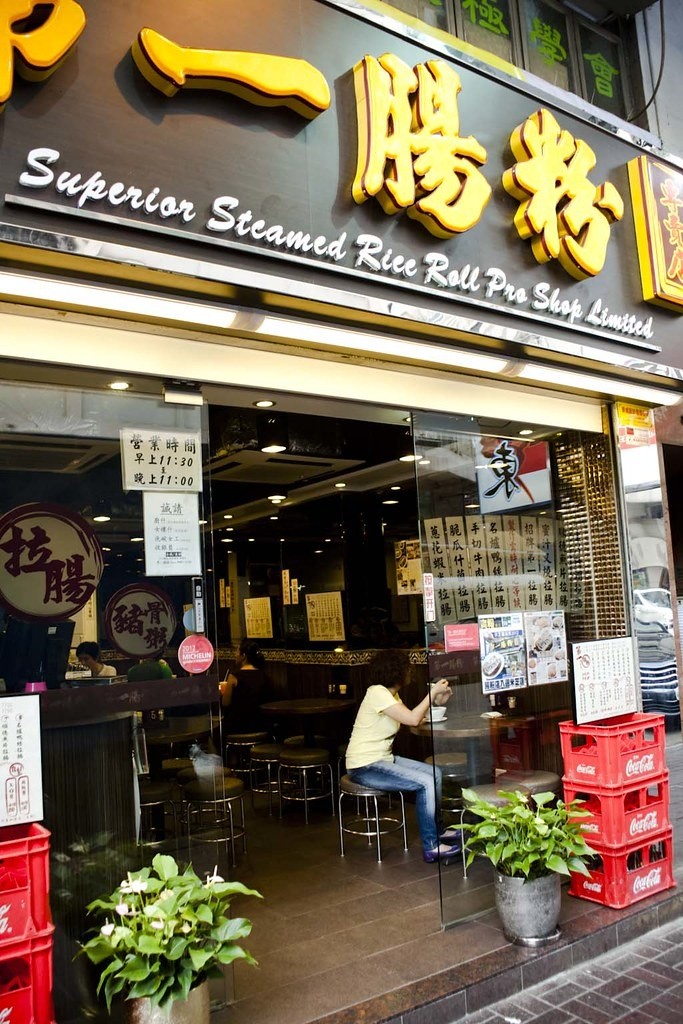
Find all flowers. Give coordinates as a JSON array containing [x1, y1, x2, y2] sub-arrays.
[[455, 780, 600, 886], [79, 851, 260, 1017]]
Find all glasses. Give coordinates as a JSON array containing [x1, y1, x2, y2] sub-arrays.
[[79, 656, 91, 662]]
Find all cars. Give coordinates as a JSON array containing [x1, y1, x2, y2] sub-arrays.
[[632, 617, 682, 716], [634, 587, 674, 635]]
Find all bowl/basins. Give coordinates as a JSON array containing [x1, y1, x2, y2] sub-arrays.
[[424, 707, 446, 718]]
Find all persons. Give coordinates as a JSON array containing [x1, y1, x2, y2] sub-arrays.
[[126, 650, 176, 683], [198, 640, 270, 762], [76, 641, 119, 678], [343, 646, 467, 864]]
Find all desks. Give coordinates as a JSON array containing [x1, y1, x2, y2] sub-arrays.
[[261, 698, 357, 794], [410, 706, 569, 784], [137, 716, 221, 812]]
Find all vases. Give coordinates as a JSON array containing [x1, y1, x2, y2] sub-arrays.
[[492, 865, 562, 938], [131, 972, 212, 1024]]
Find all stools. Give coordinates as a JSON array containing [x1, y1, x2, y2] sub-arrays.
[[249, 744, 285, 814], [138, 784, 181, 861], [497, 768, 560, 809], [426, 752, 473, 836], [188, 776, 245, 868], [461, 784, 532, 879], [224, 729, 267, 789], [335, 776, 409, 864], [162, 757, 196, 822], [178, 767, 230, 838], [279, 742, 334, 824]]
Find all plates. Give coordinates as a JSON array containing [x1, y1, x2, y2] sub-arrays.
[[482, 654, 505, 679], [424, 717, 450, 723]]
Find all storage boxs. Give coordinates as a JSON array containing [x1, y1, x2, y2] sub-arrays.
[[559, 713, 676, 909], [0, 823, 54, 1024]]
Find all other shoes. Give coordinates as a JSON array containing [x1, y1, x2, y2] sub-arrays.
[[440, 828, 462, 840], [423, 844, 461, 862]]
